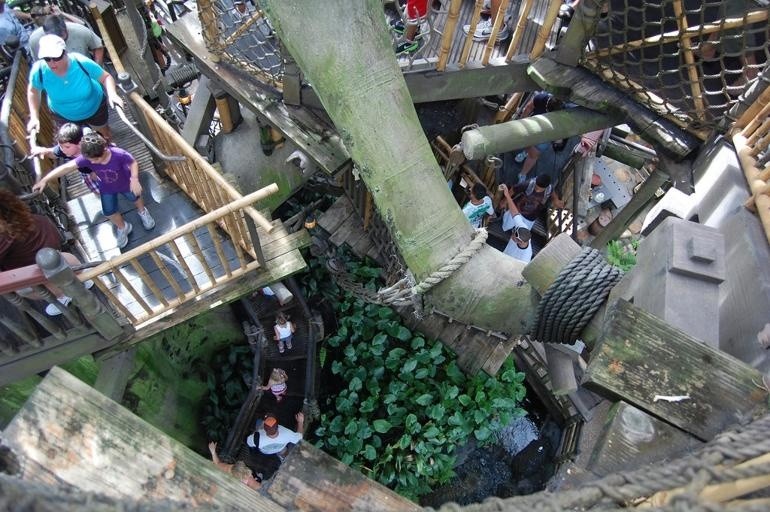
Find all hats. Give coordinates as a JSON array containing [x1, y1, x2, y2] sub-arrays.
[[37, 33, 66, 60]]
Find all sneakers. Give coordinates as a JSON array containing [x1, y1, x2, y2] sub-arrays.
[[514, 151, 528, 162], [461, 18, 509, 42], [114, 221, 135, 249], [45, 268, 96, 317], [517, 173, 526, 184], [137, 207, 158, 231], [390, 18, 422, 56]]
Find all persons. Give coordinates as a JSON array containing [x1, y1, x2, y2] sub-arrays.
[[271, 312, 298, 354], [386, 1, 514, 56], [206, 439, 264, 491], [245, 408, 304, 468], [444, 97, 639, 263], [256, 366, 288, 402]]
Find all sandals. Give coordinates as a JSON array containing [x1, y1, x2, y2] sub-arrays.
[[554, 4, 609, 21]]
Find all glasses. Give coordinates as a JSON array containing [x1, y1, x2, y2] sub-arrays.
[[514, 226, 522, 242]]
